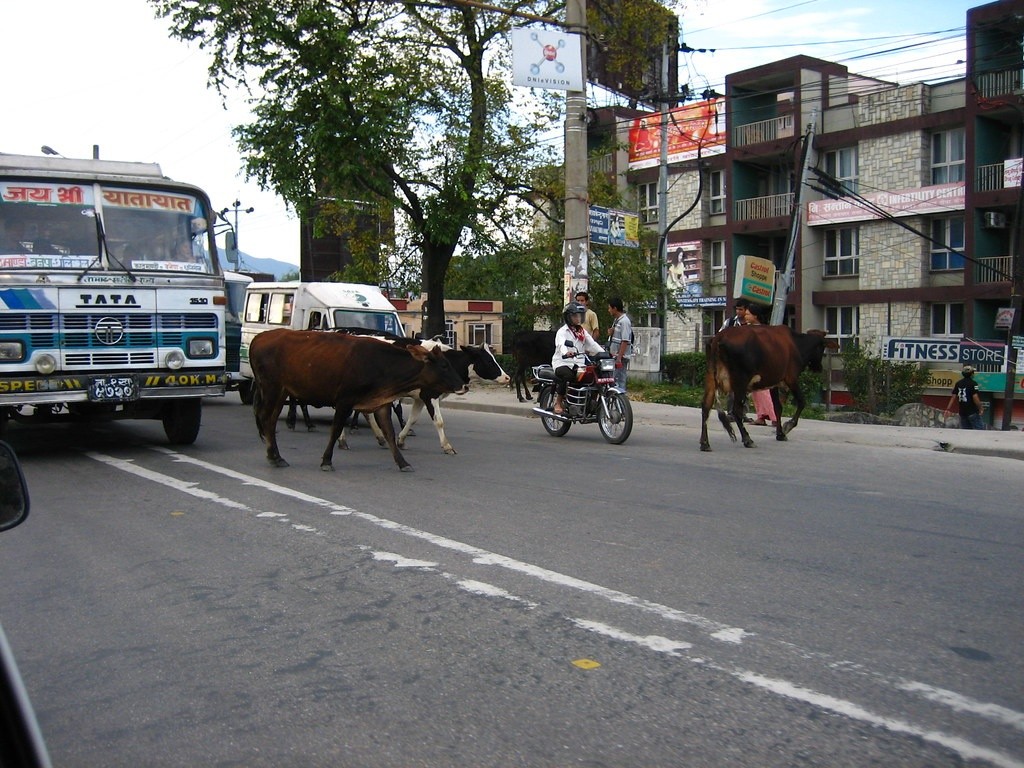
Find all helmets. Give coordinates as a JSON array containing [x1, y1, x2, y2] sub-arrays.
[[562, 301, 587, 326]]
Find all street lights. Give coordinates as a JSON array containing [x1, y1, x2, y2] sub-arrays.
[[220, 198, 255, 271]]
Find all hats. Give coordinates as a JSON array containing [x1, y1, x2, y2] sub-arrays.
[[962, 365, 977, 373]]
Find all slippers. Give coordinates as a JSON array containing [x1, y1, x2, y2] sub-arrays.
[[772, 421, 778, 426], [552, 402, 563, 414], [747, 419, 768, 426]]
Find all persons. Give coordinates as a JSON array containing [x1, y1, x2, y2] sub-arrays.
[[611, 215, 621, 239], [574, 292, 599, 365], [719, 299, 777, 426], [667, 248, 686, 298], [944, 366, 983, 430], [33, 227, 62, 254], [636, 121, 650, 150], [172, 237, 197, 262], [551, 302, 605, 416], [0, 218, 33, 254], [608, 298, 631, 390]]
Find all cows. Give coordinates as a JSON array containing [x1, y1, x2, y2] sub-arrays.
[[248, 327, 511, 473], [698, 325, 831, 452]]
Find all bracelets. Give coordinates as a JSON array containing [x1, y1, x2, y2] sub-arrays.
[[945, 408, 950, 411]]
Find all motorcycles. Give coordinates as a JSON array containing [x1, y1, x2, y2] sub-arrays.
[[531, 339, 634, 444]]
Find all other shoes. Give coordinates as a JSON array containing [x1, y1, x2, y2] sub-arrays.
[[726, 413, 735, 422], [743, 417, 754, 422]]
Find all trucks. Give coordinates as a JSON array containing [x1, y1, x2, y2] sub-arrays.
[[0, 150, 226, 444], [220, 271, 257, 404]]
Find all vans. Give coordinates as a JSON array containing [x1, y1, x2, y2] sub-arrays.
[[240, 281, 408, 379]]
